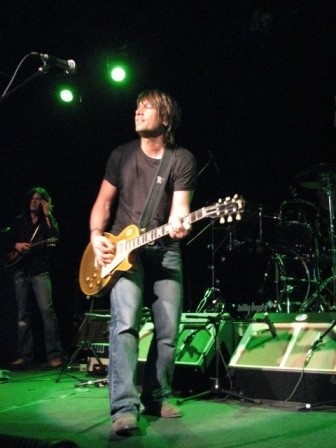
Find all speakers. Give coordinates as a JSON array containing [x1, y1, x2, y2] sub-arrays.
[[137, 319, 235, 390], [75, 309, 110, 357], [230, 322, 336, 398]]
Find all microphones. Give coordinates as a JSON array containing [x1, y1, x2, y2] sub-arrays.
[[208, 149, 221, 172], [305, 342, 316, 364], [178, 335, 193, 352], [31, 51, 77, 74], [262, 307, 277, 338]]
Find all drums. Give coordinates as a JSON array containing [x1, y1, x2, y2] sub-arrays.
[[294, 242, 336, 263], [219, 240, 310, 320], [276, 200, 321, 240]]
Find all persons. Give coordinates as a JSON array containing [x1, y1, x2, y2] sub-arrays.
[[4, 187, 62, 367], [90, 90, 198, 435]]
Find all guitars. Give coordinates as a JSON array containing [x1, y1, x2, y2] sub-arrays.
[[78, 194, 246, 298], [3, 234, 59, 269]]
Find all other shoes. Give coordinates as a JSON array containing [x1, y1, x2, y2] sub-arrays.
[[112, 416, 138, 434], [50, 357, 61, 366], [143, 399, 182, 417], [13, 358, 28, 366]]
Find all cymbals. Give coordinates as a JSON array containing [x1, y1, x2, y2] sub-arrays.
[[295, 163, 336, 190]]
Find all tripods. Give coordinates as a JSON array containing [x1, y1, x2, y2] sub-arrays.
[[174, 224, 260, 406]]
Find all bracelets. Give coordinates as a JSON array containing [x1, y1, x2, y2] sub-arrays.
[[91, 228, 104, 233]]
